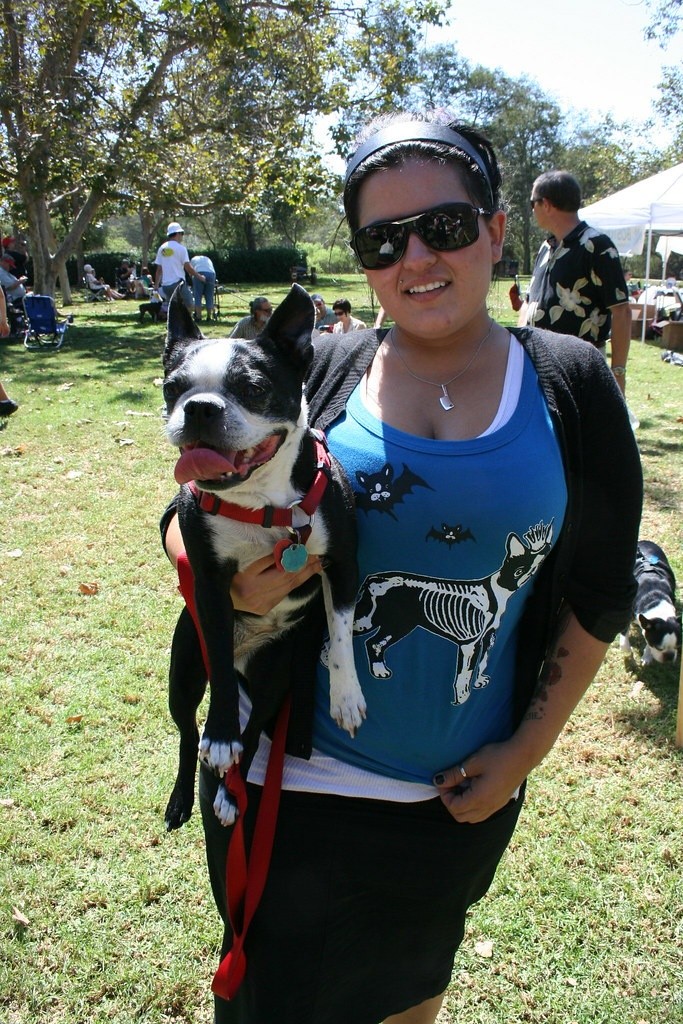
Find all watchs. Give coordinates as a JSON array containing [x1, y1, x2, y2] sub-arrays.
[[610, 366, 627, 377]]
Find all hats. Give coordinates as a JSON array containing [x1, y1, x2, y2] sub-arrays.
[[312, 294, 323, 302], [2, 238, 14, 247], [2, 254, 17, 269], [122, 259, 130, 266], [167, 222, 184, 235]]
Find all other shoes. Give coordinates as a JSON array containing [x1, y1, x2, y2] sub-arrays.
[[0, 399, 18, 415]]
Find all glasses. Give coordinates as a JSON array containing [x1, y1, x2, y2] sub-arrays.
[[531, 198, 558, 209], [335, 311, 345, 316], [315, 303, 321, 307], [349, 202, 495, 270]]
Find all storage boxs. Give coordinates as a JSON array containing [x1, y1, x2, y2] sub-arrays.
[[629, 304, 655, 338], [653, 320, 683, 349]]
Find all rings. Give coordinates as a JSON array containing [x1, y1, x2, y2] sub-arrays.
[[460, 763, 467, 778]]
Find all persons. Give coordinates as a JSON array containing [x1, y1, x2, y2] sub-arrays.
[[311, 292, 338, 333], [2, 235, 30, 290], [622, 265, 639, 304], [140, 266, 155, 288], [153, 221, 206, 317], [331, 298, 366, 336], [0, 252, 29, 311], [161, 115, 645, 1024], [119, 257, 137, 289], [228, 297, 272, 340], [188, 254, 217, 322], [129, 279, 146, 300], [517, 165, 633, 396], [83, 264, 126, 301], [0, 285, 18, 416]]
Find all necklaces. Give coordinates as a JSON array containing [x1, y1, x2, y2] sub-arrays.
[[390, 316, 495, 410]]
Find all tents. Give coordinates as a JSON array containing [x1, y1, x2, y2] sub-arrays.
[[578, 160, 683, 284]]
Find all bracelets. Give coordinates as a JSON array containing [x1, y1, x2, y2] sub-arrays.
[[17, 279, 21, 284]]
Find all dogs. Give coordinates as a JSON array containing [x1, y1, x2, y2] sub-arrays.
[[139, 297, 165, 323], [620, 541, 680, 667], [158, 280, 366, 833]]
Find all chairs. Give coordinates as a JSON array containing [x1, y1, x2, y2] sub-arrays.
[[1, 284, 73, 349], [114, 267, 134, 299], [82, 277, 105, 302]]
